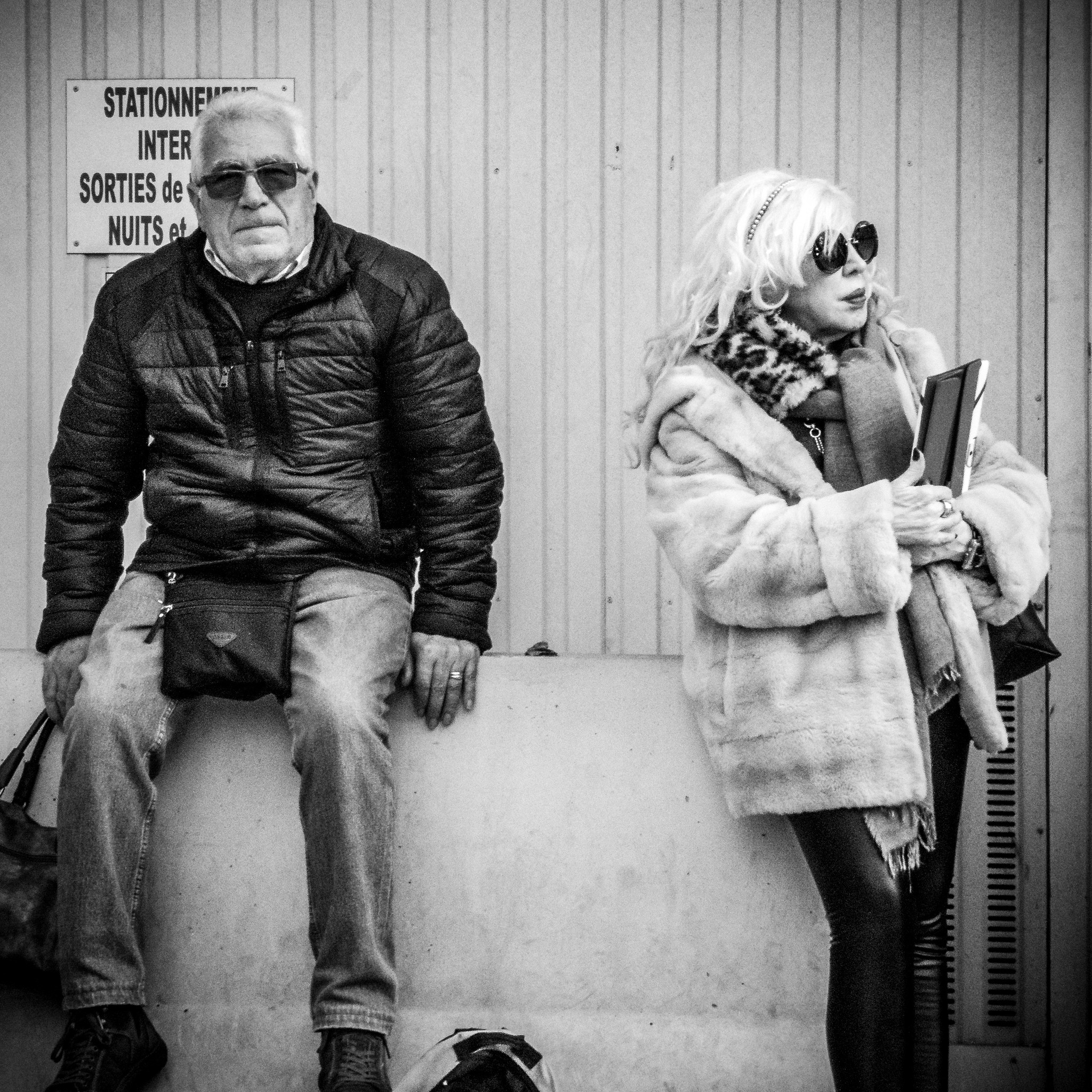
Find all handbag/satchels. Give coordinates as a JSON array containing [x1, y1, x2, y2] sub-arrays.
[[0, 707, 64, 1008], [144, 568, 309, 701], [986, 599, 1061, 688]]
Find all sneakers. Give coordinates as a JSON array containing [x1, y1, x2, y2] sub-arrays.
[[317, 1026, 394, 1092], [44, 1003, 169, 1092]]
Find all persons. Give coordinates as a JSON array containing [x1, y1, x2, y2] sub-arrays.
[[449, 672, 463, 679], [625, 168, 1050, 1092], [32, 88, 505, 1092]]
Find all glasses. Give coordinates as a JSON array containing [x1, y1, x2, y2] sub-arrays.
[[805, 220, 879, 275], [196, 162, 307, 202]]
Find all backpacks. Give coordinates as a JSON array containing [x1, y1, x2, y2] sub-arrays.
[[393, 1026, 562, 1092]]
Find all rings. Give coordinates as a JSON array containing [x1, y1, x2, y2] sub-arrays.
[[938, 499, 953, 519]]
